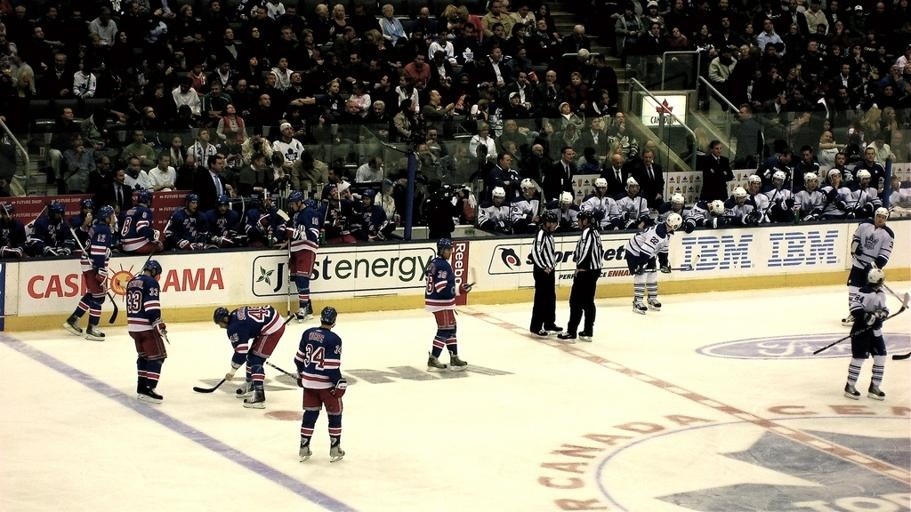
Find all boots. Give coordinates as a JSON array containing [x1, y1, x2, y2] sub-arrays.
[[243, 387, 265, 403], [631, 296, 649, 311], [291, 306, 313, 319], [844, 382, 862, 395], [427, 353, 448, 369], [136, 381, 164, 399], [329, 444, 346, 457], [530, 323, 593, 339], [67, 313, 83, 333], [299, 445, 313, 457], [868, 381, 886, 396], [841, 314, 856, 323], [647, 294, 663, 307], [236, 378, 254, 394], [450, 355, 467, 366], [85, 323, 105, 338]]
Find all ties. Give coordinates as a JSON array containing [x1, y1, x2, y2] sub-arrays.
[[649, 166, 655, 182], [117, 190, 123, 210]]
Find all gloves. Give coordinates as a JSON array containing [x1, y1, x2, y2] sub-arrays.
[[152, 319, 166, 337], [95, 268, 109, 284], [296, 372, 304, 388], [660, 262, 672, 275], [331, 379, 348, 399], [865, 309, 889, 330], [454, 282, 471, 297]]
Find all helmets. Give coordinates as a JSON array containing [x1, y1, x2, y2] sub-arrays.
[[876, 207, 889, 217], [137, 192, 151, 207], [217, 195, 229, 204], [491, 177, 640, 208], [732, 170, 871, 198], [867, 267, 887, 287], [258, 192, 375, 204], [96, 205, 114, 218], [672, 193, 685, 205], [213, 306, 229, 324], [81, 198, 94, 217], [48, 200, 65, 224], [436, 236, 456, 249], [185, 194, 199, 201], [577, 211, 593, 219], [667, 212, 682, 227], [710, 200, 724, 215], [2, 201, 15, 223], [142, 260, 164, 276], [544, 211, 558, 222], [319, 306, 338, 325]]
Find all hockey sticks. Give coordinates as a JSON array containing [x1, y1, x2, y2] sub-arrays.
[[891, 352, 911, 361], [69, 229, 118, 325], [644, 255, 699, 273], [193, 310, 298, 395], [814, 293, 908, 355], [285, 237, 291, 325], [464, 267, 477, 288]]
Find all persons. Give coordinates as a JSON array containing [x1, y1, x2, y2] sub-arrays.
[[280, 190, 322, 324], [62, 205, 119, 342], [625, 213, 684, 312], [528, 211, 565, 338], [558, 209, 604, 340], [212, 304, 288, 410], [2, 2, 911, 257], [423, 238, 470, 373], [845, 268, 891, 400], [842, 207, 895, 324], [125, 258, 169, 403], [292, 306, 349, 458]]
